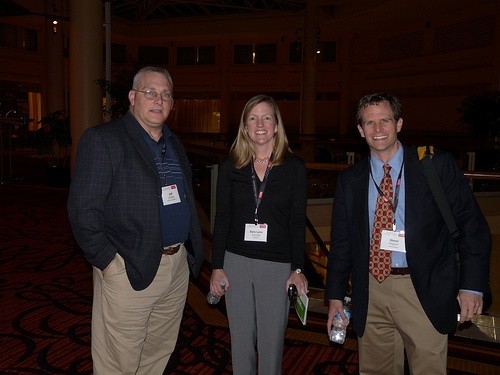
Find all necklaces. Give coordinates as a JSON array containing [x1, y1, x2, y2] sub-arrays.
[[254, 155, 271, 164]]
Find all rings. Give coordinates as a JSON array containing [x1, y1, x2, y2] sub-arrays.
[[473, 312, 477, 315]]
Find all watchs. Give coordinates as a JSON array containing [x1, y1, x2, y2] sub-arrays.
[[291, 268, 304, 274]]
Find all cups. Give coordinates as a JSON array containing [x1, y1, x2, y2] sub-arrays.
[[467, 152, 475, 170], [347, 153, 354, 165]]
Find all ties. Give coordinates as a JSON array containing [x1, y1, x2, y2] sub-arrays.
[[369, 164, 392, 284]]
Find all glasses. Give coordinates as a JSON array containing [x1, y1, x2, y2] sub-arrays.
[[133, 89, 173, 101]]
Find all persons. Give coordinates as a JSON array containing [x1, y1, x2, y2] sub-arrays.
[[67, 67, 203, 375], [210, 95, 309, 375], [325, 92, 490, 375]]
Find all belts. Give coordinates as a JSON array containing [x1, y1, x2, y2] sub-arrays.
[[390, 267, 410, 275], [162, 245, 181, 255]]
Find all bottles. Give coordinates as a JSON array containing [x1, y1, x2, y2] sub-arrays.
[[329, 297, 353, 343], [207, 280, 226, 304], [288, 285, 298, 301]]
